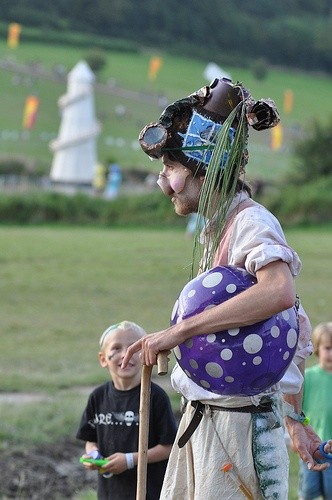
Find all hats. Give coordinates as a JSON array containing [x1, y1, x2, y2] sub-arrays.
[[138, 77, 280, 181]]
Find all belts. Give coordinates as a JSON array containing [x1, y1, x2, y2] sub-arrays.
[[178, 401, 273, 448]]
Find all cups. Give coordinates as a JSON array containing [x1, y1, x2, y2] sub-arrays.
[[88, 450, 113, 478]]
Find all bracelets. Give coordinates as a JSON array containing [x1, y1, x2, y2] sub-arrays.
[[125, 452, 134, 470], [284, 400, 311, 427]]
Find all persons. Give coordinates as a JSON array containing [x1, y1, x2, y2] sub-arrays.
[[90, 156, 160, 202], [283, 322, 332, 500], [75, 320, 178, 500], [119, 77, 313, 500]]
